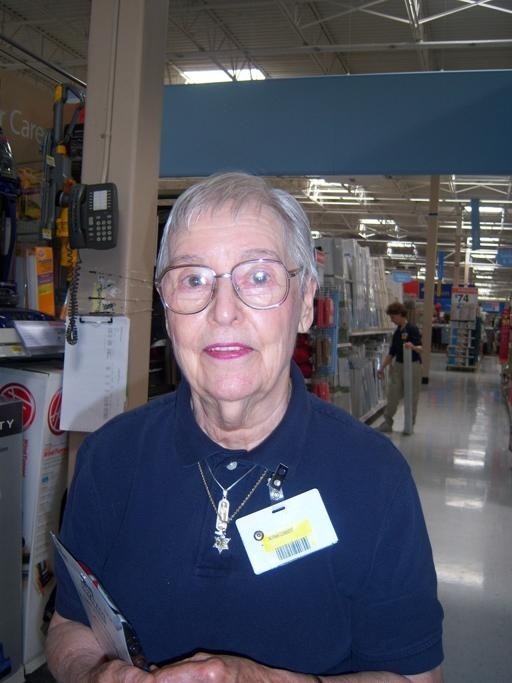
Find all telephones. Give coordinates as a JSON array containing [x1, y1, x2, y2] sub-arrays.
[[67, 182, 119, 250]]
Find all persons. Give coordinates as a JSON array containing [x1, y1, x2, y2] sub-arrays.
[[373, 304, 424, 436], [42, 169, 444, 683]]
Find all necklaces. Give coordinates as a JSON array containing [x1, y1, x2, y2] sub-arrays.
[[196, 462, 269, 555], [204, 458, 259, 533]]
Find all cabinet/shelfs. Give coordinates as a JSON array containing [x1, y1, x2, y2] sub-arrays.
[[314, 234, 480, 430]]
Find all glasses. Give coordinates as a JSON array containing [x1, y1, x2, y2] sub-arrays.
[[154, 259, 303, 315]]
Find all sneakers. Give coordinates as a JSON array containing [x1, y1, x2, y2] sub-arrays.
[[375, 422, 392, 432]]
